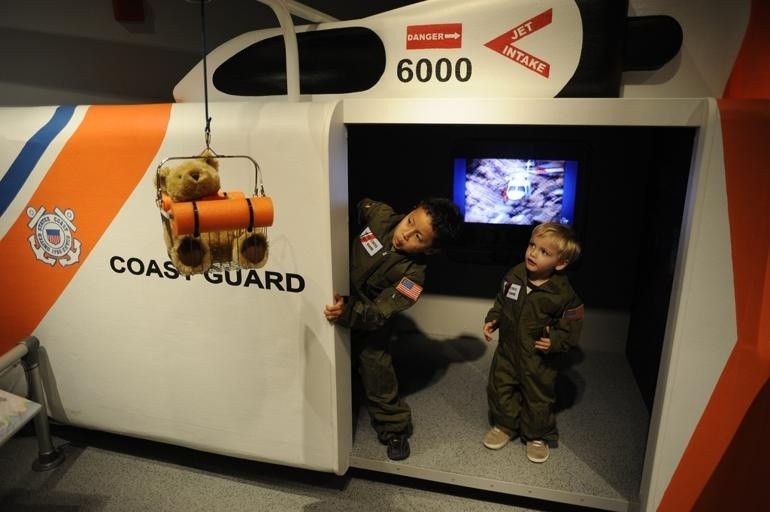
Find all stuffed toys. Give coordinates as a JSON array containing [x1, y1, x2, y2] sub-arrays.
[[153, 147, 270, 276]]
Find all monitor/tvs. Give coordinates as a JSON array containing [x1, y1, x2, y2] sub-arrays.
[[448, 151, 585, 233]]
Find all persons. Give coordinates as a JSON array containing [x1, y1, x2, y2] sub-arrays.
[[324, 196, 465, 461], [482, 223, 583, 463]]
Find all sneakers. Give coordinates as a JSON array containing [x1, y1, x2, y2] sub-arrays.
[[386, 434, 412, 462], [525, 438, 551, 464], [482, 425, 512, 451]]
